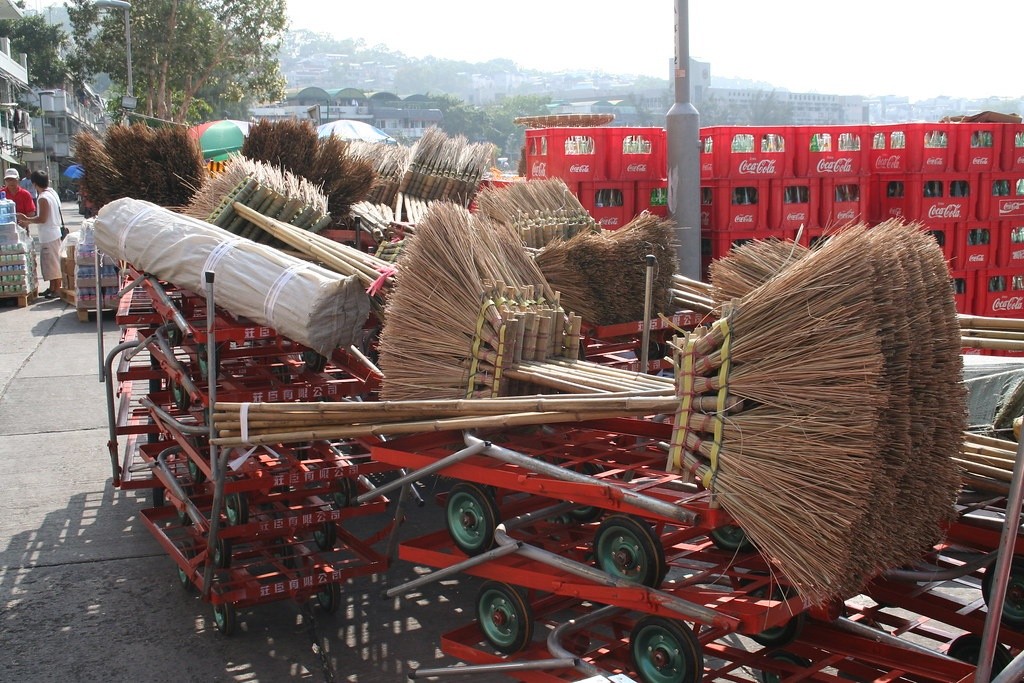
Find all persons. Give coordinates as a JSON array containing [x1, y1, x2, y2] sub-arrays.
[[20, 169, 38, 216], [0, 168, 36, 237], [16, 170, 63, 300]]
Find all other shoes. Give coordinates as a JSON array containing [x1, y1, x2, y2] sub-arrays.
[[38, 288, 52, 296], [45, 291, 60, 299]]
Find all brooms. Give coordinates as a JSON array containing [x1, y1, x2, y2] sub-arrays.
[[65, 115, 497, 305], [208, 174, 1020, 631]]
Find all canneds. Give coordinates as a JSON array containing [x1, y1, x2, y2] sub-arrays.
[[0, 242, 36, 292]]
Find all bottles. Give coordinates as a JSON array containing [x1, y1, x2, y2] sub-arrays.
[[76, 244, 118, 301], [0, 191, 17, 224], [531, 122, 1024, 293]]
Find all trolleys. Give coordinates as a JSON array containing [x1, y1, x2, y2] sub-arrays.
[[88, 247, 1024, 683]]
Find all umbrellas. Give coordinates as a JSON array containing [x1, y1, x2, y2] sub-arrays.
[[315, 119, 397, 144], [187, 120, 260, 161], [64, 165, 86, 179]]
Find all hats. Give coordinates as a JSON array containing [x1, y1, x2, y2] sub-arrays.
[[4, 168, 19, 180]]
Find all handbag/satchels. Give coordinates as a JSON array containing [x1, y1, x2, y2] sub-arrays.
[[60, 227, 69, 240]]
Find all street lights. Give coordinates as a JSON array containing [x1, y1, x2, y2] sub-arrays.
[[91, 0, 135, 117], [38, 90, 55, 185], [93, 121, 105, 134]]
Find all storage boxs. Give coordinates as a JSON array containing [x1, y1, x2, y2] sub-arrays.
[[60, 246, 75, 290], [524, 122, 1024, 353], [0, 233, 18, 243], [0, 223, 16, 233]]
